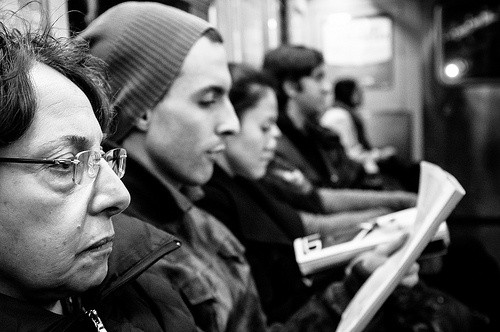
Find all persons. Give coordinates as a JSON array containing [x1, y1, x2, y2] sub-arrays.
[[193, 44, 451, 327], [78, 2, 419, 332], [1, 28, 197, 332]]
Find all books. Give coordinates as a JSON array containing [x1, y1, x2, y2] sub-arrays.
[[336, 161, 465, 332], [294, 206, 449, 277]]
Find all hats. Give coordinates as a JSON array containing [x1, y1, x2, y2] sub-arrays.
[[75, 1, 217, 141]]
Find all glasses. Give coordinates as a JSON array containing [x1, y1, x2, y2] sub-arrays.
[[0, 139, 127, 185]]
